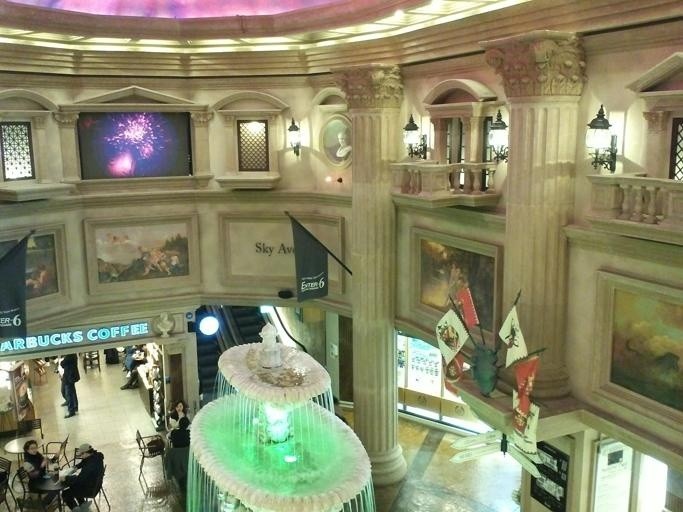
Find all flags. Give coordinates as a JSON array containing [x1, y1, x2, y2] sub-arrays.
[[511, 398, 543, 465], [513, 356, 541, 417], [291, 217, 328, 304], [497, 304, 530, 368], [444, 285, 481, 327], [434, 306, 472, 364]]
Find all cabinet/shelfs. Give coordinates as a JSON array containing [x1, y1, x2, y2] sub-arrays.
[[149, 344, 181, 432]]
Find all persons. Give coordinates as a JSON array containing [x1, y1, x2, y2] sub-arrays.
[[448, 259, 467, 306], [23, 437, 50, 480], [53, 344, 191, 512], [335, 129, 350, 157], [26, 263, 49, 292], [138, 246, 172, 275]]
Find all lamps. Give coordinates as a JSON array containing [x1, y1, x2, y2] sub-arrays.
[[583, 103, 619, 172], [401, 113, 429, 160], [488, 108, 511, 164], [286, 114, 302, 156]]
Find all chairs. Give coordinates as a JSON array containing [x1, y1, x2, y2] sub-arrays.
[[82, 351, 101, 373], [0, 418, 111, 512], [133, 428, 170, 483]]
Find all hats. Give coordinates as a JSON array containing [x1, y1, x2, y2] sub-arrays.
[[76, 443, 92, 455]]
